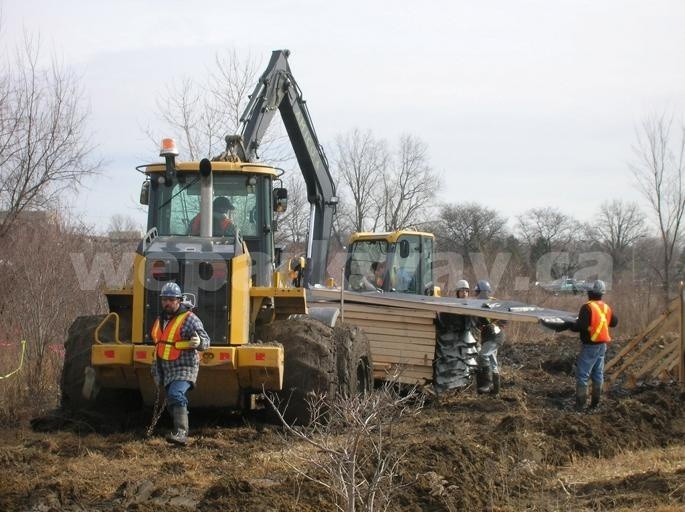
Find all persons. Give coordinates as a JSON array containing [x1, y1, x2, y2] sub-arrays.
[[151, 280, 210, 445], [473, 280, 507, 394], [570, 279, 618, 410], [186, 196, 241, 236], [455, 278, 469, 298], [369, 261, 398, 289]]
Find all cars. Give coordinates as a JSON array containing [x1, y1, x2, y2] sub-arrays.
[[540, 275, 587, 297]]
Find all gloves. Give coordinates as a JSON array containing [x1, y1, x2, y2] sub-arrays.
[[190, 330, 201, 348]]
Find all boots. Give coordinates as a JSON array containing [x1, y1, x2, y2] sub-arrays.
[[167, 406, 189, 445], [491, 373, 500, 395], [575, 384, 588, 409], [590, 384, 603, 408], [478, 366, 494, 392]]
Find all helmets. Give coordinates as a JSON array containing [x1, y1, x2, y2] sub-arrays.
[[159, 282, 183, 298], [454, 280, 470, 292], [474, 280, 493, 294], [587, 279, 607, 295]]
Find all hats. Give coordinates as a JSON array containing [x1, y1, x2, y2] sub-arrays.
[[213, 196, 235, 209]]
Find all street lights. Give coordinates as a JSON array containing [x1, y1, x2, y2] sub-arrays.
[[629, 235, 647, 285]]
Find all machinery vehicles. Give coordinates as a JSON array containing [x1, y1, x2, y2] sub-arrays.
[[325, 230, 442, 297], [59, 50, 372, 429]]
